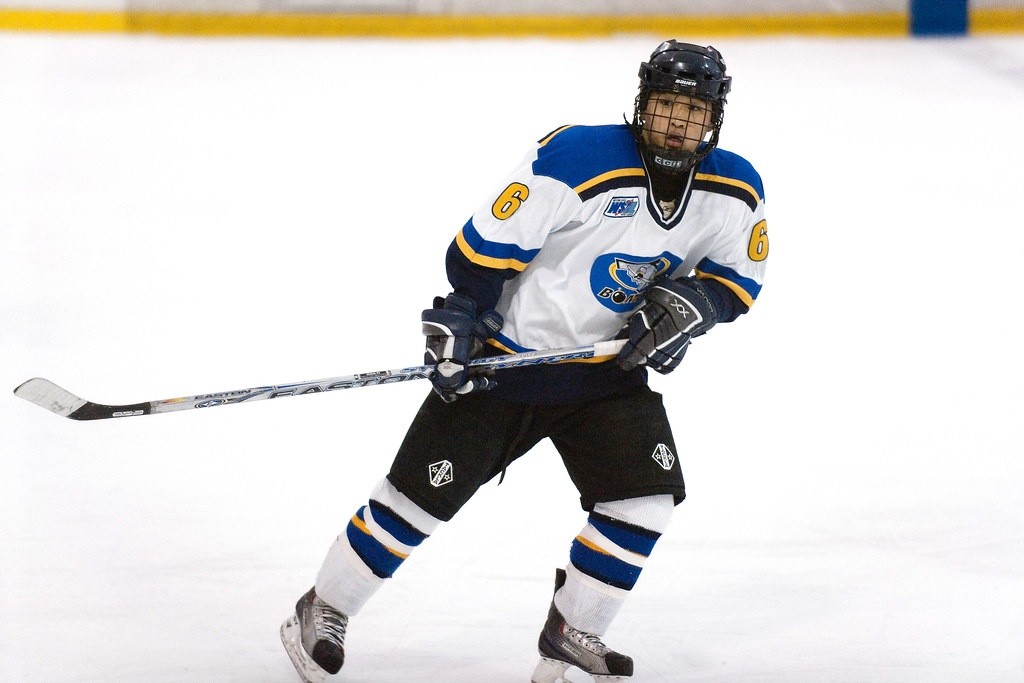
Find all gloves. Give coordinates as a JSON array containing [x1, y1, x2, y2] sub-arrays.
[[616, 275, 717, 375], [420, 293, 503, 406]]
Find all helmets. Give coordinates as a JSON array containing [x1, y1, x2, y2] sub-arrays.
[[633, 39, 732, 174]]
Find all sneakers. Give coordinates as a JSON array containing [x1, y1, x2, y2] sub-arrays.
[[531, 568, 634, 683], [280, 585, 348, 683]]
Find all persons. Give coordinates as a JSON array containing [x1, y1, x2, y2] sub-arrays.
[[272, 39, 771, 681]]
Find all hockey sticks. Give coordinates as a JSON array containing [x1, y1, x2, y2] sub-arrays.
[[9, 336, 629, 422]]
